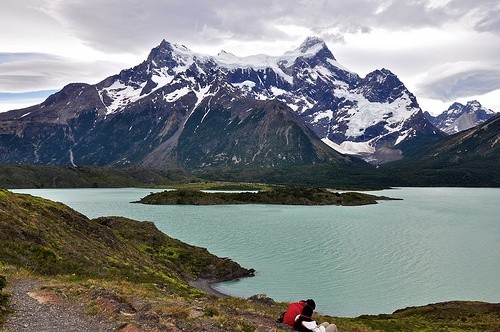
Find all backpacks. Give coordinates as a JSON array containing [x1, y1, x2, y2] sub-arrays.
[[293, 315, 318, 332], [282, 300, 308, 326]]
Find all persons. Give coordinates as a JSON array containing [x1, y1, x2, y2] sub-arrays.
[[293, 306, 338, 332], [283, 297, 316, 328]]
